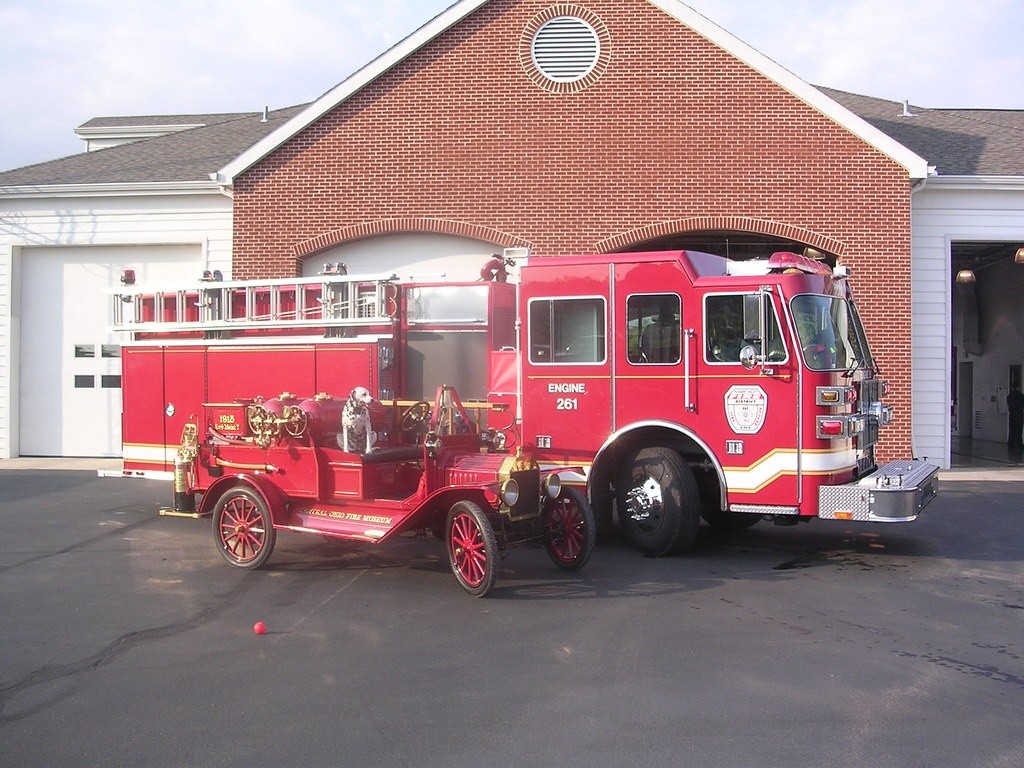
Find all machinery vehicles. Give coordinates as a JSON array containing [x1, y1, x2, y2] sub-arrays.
[[156, 383, 596, 601]]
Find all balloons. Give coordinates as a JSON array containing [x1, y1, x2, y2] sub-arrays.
[[254, 621, 267, 634]]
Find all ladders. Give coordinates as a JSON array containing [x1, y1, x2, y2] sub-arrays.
[[110, 275, 398, 334]]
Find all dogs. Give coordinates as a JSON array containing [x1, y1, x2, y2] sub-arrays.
[[341, 386, 382, 454]]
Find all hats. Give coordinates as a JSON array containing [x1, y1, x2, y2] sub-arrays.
[[801, 317, 814, 324]]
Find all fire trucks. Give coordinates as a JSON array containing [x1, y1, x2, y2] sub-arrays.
[[109, 245, 942, 560]]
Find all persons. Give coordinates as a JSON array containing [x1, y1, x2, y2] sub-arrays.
[[1007, 383, 1024, 449]]
